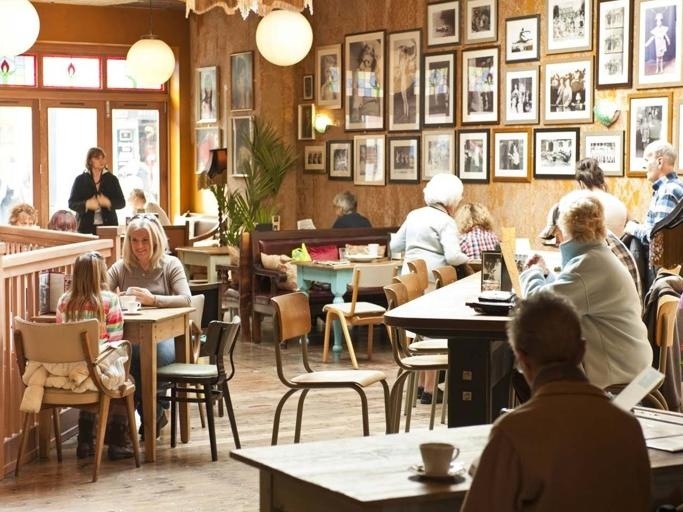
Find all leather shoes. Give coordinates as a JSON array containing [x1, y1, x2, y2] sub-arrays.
[[76, 442, 96, 458], [107, 446, 135, 461]]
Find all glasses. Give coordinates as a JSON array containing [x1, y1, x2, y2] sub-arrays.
[[130, 214, 161, 232]]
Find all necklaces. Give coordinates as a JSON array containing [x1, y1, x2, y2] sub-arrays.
[[93, 180, 101, 185], [140, 263, 146, 277]]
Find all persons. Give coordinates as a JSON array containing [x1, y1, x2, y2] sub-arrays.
[[303, 10, 671, 173], [456, 289, 654, 511], [0, 188, 15, 213], [509, 188, 654, 403], [6, 204, 40, 229], [54, 250, 142, 460], [329, 189, 373, 227], [47, 208, 80, 234], [94, 211, 194, 442], [387, 173, 473, 406], [452, 201, 502, 264], [127, 187, 170, 226], [622, 138, 683, 287], [69, 147, 126, 236], [199, 73, 216, 122], [233, 58, 251, 108], [553, 158, 630, 245]]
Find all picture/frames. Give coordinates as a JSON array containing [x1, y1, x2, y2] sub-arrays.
[[229, 115, 256, 178], [194, 129, 222, 178], [193, 65, 220, 125], [228, 50, 254, 112]]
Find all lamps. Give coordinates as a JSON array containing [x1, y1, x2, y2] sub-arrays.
[[125, 1, 179, 91], [254, 0, 316, 68], [193, 148, 231, 247], [2, 0, 42, 58]]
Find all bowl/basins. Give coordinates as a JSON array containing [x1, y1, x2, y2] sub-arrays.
[[477, 290, 511, 303]]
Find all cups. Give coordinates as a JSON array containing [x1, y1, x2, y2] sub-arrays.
[[367, 243, 379, 257], [418, 442, 459, 475], [125, 301, 141, 312], [119, 295, 136, 310], [338, 247, 351, 264]]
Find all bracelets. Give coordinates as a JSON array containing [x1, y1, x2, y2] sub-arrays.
[[151, 294, 157, 306]]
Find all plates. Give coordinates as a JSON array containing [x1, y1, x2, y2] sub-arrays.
[[345, 253, 382, 263], [464, 301, 514, 316], [118, 310, 142, 315], [408, 466, 466, 479]]
